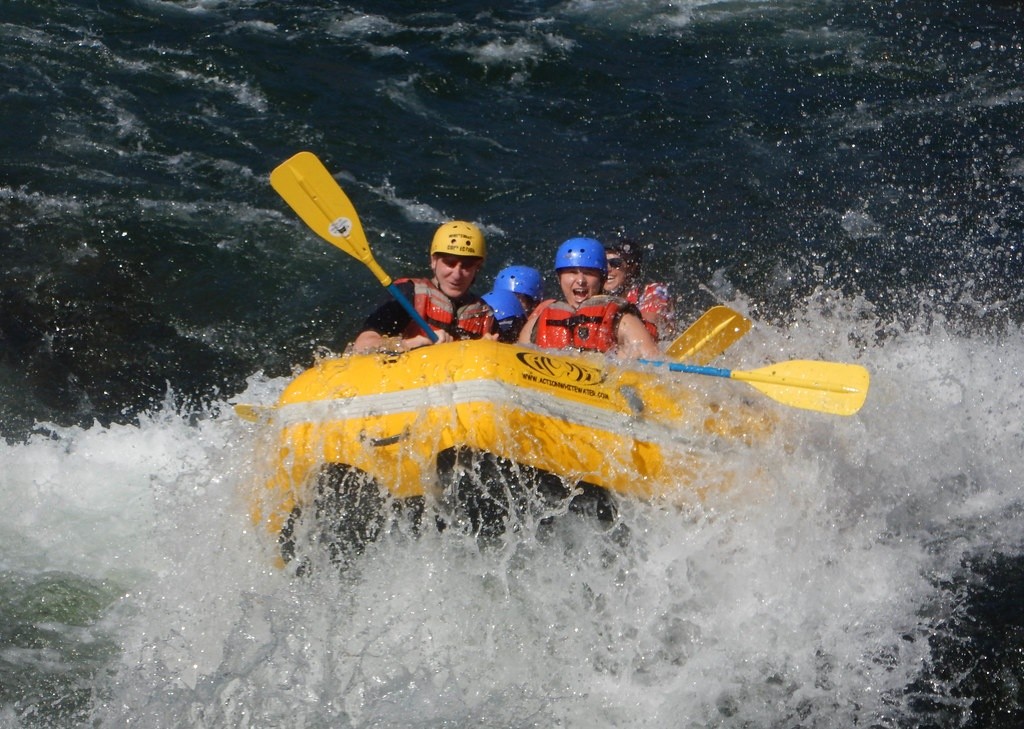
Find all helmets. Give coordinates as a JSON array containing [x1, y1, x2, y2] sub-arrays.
[[430, 220, 487, 261], [480, 289, 528, 336], [554, 237, 608, 273], [604, 237, 639, 266], [493, 265, 547, 301]]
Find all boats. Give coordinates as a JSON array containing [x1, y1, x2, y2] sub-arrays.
[[250, 336, 854, 578]]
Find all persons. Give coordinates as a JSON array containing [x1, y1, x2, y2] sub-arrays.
[[352, 221, 675, 360]]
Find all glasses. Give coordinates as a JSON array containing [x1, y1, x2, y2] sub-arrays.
[[607, 257, 624, 268], [442, 255, 478, 271]]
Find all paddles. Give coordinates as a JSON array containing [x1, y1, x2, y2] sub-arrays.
[[266, 148, 441, 343], [618, 356, 874, 417], [660, 306, 755, 376], [232, 401, 274, 423]]
